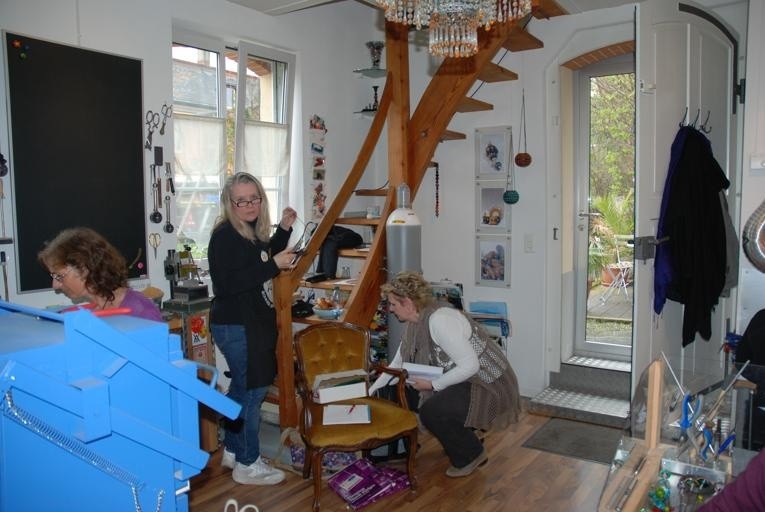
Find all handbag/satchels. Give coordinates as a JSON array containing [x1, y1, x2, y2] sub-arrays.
[[364, 382, 421, 462], [289, 431, 363, 478]]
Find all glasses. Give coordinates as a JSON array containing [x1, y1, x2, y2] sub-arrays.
[[230, 197, 262, 208], [49, 265, 81, 281]]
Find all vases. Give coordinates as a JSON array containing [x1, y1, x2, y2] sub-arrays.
[[365, 40, 384, 68]]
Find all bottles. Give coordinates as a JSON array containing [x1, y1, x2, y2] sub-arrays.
[[342, 266, 350, 278], [330, 286, 339, 303]]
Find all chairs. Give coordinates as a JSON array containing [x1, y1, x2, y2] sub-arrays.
[[293, 321, 423, 512], [597, 225, 633, 309]]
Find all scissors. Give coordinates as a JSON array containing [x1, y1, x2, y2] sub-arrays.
[[225, 497, 259, 511], [145, 110, 160, 151], [160, 104, 172, 136], [149, 233, 161, 260]]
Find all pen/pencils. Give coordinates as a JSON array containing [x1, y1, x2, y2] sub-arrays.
[[348, 404, 355, 415]]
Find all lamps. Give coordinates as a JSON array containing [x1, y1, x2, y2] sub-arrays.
[[375, 0, 537, 64]]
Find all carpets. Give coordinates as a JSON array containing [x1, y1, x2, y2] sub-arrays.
[[518, 416, 630, 465]]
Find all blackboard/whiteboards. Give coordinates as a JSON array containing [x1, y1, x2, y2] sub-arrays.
[[2, 29, 149, 294]]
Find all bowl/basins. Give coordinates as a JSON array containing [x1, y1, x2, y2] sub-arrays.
[[312, 303, 342, 320]]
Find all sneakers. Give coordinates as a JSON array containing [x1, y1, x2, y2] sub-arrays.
[[221, 447, 286, 485], [446, 449, 489, 477]]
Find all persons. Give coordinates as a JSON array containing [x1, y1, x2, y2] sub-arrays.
[[481, 243, 506, 280], [209, 170, 298, 487], [37, 225, 161, 321], [369, 269, 522, 477]]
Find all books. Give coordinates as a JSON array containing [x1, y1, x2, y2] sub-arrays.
[[314, 374, 369, 403], [401, 361, 446, 386]]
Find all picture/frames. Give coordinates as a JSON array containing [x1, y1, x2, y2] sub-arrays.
[[475, 236, 512, 289], [473, 181, 513, 235], [474, 125, 513, 180]]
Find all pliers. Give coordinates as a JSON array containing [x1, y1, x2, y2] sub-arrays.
[[677, 390, 704, 444]]
